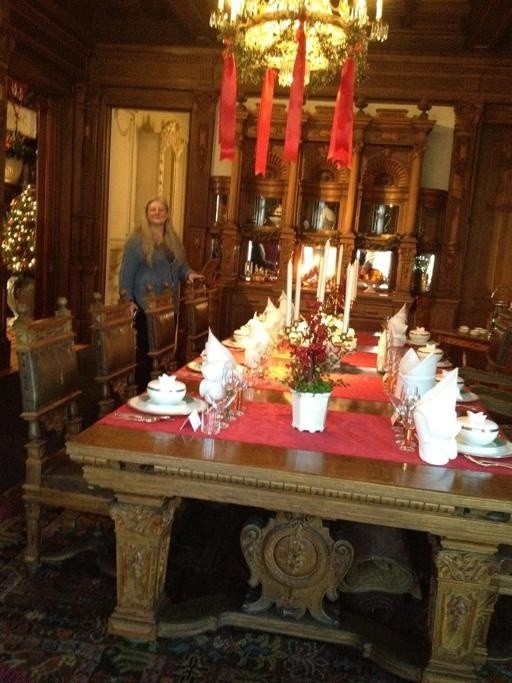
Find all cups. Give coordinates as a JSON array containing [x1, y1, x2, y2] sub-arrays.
[[395, 385, 418, 440], [244, 359, 257, 387], [398, 392, 417, 447], [215, 387, 229, 429], [201, 391, 220, 434], [235, 364, 246, 416], [222, 360, 237, 423], [400, 399, 416, 452]]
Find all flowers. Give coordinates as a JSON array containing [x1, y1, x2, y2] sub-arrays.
[[286, 314, 357, 357], [276, 307, 333, 392]]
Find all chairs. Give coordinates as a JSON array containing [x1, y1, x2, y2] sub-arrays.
[[144, 281, 178, 380], [483, 398, 512, 424], [458, 367, 512, 388], [184, 278, 210, 359], [89, 290, 138, 418], [8, 296, 116, 577], [499, 557, 512, 595], [500, 425, 512, 441]]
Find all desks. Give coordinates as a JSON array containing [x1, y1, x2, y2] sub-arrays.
[[222, 279, 413, 337]]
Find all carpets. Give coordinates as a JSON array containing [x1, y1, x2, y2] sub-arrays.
[[0, 487, 511, 681]]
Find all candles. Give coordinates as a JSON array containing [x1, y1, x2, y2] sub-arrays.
[[248, 240, 252, 262], [351, 258, 357, 301], [286, 251, 293, 333], [342, 265, 353, 340], [294, 253, 302, 325], [355, 249, 360, 294], [337, 244, 344, 289], [346, 262, 350, 319], [319, 239, 330, 302], [317, 258, 323, 301]]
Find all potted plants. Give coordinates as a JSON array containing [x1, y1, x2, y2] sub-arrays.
[[415, 255, 430, 295], [4, 129, 26, 185]]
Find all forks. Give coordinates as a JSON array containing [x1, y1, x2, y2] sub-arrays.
[[463, 454, 512, 466], [468, 456, 512, 469]]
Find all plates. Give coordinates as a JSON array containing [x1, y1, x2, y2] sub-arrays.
[[407, 339, 439, 347], [223, 338, 241, 349], [455, 392, 478, 403], [187, 360, 203, 372], [436, 360, 452, 367], [456, 440, 512, 459], [126, 395, 206, 416]]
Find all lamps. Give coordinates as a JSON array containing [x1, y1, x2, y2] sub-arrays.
[[210, 0, 389, 177]]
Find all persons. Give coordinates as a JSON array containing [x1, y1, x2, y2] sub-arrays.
[[119, 197, 205, 394]]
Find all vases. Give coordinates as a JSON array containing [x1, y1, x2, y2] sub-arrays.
[[326, 353, 340, 370], [290, 390, 331, 434]]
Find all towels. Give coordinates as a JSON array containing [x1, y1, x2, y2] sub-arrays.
[[262, 295, 279, 326], [400, 348, 420, 381], [239, 317, 270, 353], [412, 368, 462, 438], [411, 351, 438, 385], [278, 291, 295, 318], [200, 326, 238, 401], [386, 302, 407, 346]]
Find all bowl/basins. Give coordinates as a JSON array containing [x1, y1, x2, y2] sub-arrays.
[[435, 373, 465, 392], [233, 330, 249, 343], [471, 330, 480, 337], [459, 326, 469, 333], [479, 330, 489, 336], [409, 330, 431, 342], [416, 347, 443, 362], [457, 416, 499, 444], [146, 380, 186, 403]]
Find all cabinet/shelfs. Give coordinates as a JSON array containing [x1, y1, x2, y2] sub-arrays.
[[221, 94, 437, 292]]
